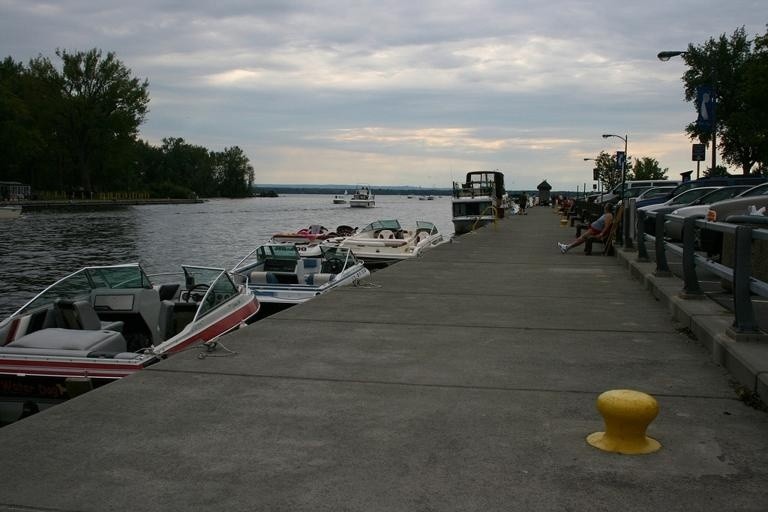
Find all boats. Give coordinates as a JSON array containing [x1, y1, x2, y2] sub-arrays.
[[407, 195, 442, 200], [333, 194, 347, 204], [343, 190, 349, 195], [349, 185, 376, 208], [451, 170, 508, 235]]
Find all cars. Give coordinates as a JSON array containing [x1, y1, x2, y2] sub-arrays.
[[583, 179, 768, 257]]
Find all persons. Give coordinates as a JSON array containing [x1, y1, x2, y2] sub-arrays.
[[518, 191, 527, 215], [557, 203, 614, 254], [551, 194, 576, 217]]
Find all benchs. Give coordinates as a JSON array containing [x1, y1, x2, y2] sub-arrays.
[[565, 201, 620, 256]]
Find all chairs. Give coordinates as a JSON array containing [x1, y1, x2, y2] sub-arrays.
[[53, 298, 125, 334], [377, 229, 396, 239], [243, 266, 336, 283]]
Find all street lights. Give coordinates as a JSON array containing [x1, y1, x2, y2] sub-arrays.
[[602, 133, 628, 247], [657, 49, 717, 178], [584, 158, 611, 193]]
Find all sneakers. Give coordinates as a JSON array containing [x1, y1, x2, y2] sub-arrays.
[[557, 242, 569, 255]]
